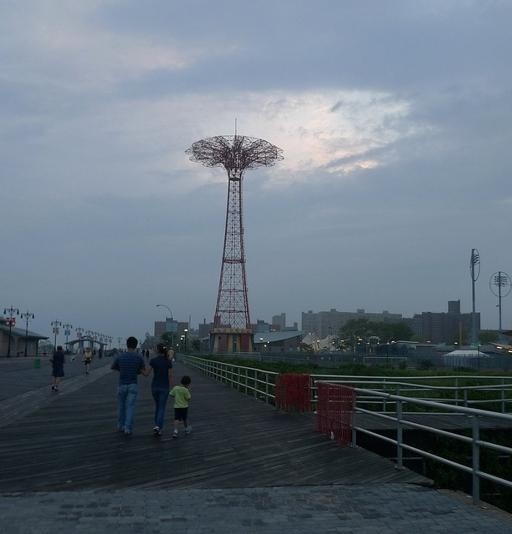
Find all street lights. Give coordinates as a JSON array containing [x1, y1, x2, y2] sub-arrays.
[[312, 338, 396, 358], [3, 305, 19, 356], [477, 343, 481, 368], [176, 329, 187, 354], [49, 320, 113, 356], [21, 308, 35, 355], [156, 304, 174, 346]]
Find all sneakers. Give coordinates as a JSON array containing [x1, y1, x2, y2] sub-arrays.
[[172, 430, 178, 438], [51, 385, 58, 391], [153, 425, 162, 436], [117, 427, 133, 435], [185, 424, 192, 435]]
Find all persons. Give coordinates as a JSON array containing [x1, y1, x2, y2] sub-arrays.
[[49, 345, 64, 391], [140, 342, 173, 435], [169, 375, 192, 438], [81, 346, 92, 375], [110, 337, 145, 434], [92, 346, 175, 365]]
